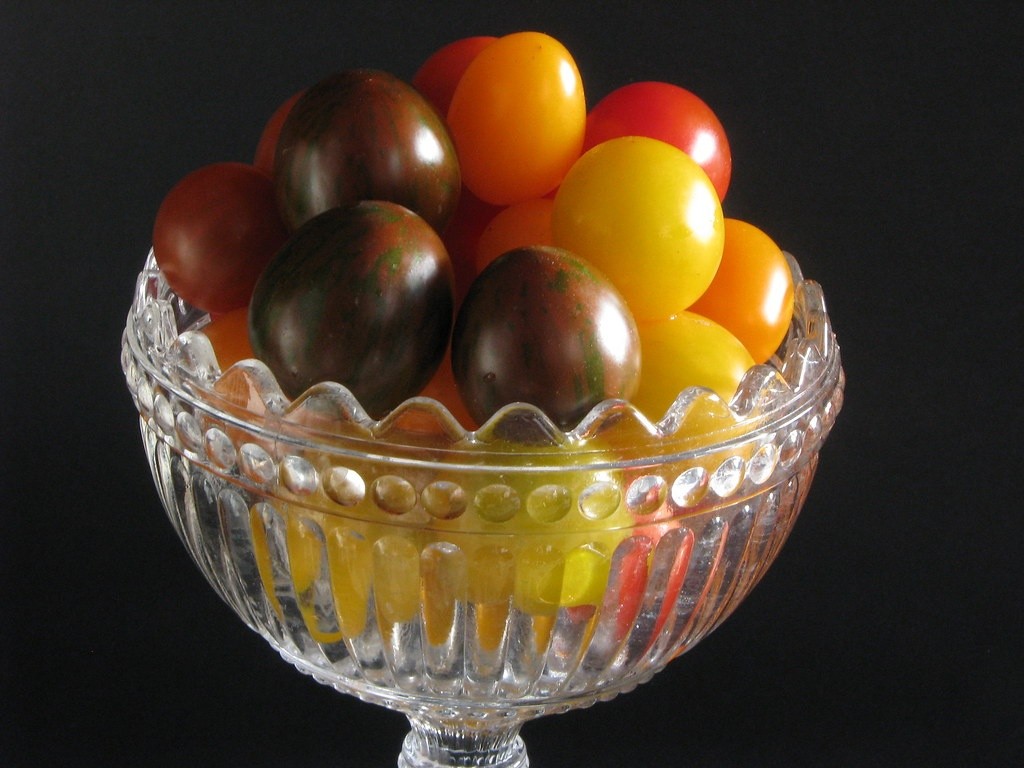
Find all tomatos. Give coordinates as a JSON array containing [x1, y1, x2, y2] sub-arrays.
[[155, 31, 794, 648]]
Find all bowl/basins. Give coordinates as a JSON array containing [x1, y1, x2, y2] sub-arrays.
[[121, 246, 845, 768]]
